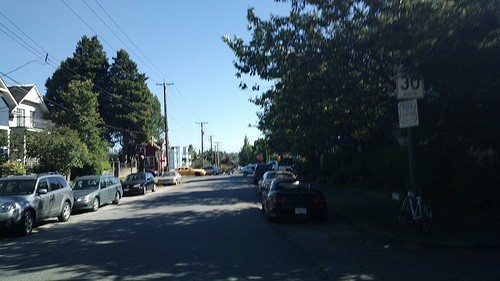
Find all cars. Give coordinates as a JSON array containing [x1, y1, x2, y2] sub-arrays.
[[158, 171, 181, 186], [240, 164, 256, 177], [257, 171, 296, 196], [124, 172, 156, 194], [204, 167, 224, 175], [262, 178, 327, 225], [176, 165, 207, 177], [70, 175, 123, 210]]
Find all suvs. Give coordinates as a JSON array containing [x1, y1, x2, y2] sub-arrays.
[[0, 172, 74, 236]]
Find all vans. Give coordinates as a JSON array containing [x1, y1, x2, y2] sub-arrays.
[[253, 163, 272, 183]]
